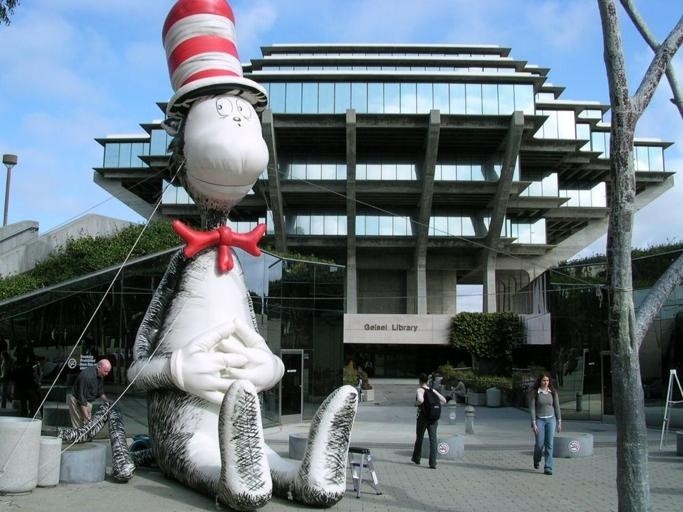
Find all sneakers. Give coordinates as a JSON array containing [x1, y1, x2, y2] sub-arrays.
[[534, 463, 539, 469], [545, 471, 552, 475], [430, 465, 436, 469], [412, 458, 420, 464]]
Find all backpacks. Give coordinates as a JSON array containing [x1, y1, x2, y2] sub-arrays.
[[425, 389, 441, 420]]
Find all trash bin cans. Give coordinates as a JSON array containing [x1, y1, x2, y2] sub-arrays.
[[486, 387, 502, 407]]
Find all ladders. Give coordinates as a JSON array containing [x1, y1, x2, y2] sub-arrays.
[[347, 447, 383, 499], [659, 368, 683, 458]]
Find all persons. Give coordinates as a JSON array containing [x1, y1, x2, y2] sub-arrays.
[[529, 371, 561, 474], [67, 358, 112, 429], [409, 371, 447, 468], [0, 339, 44, 419], [448, 377, 466, 400], [429, 370, 436, 390]]
